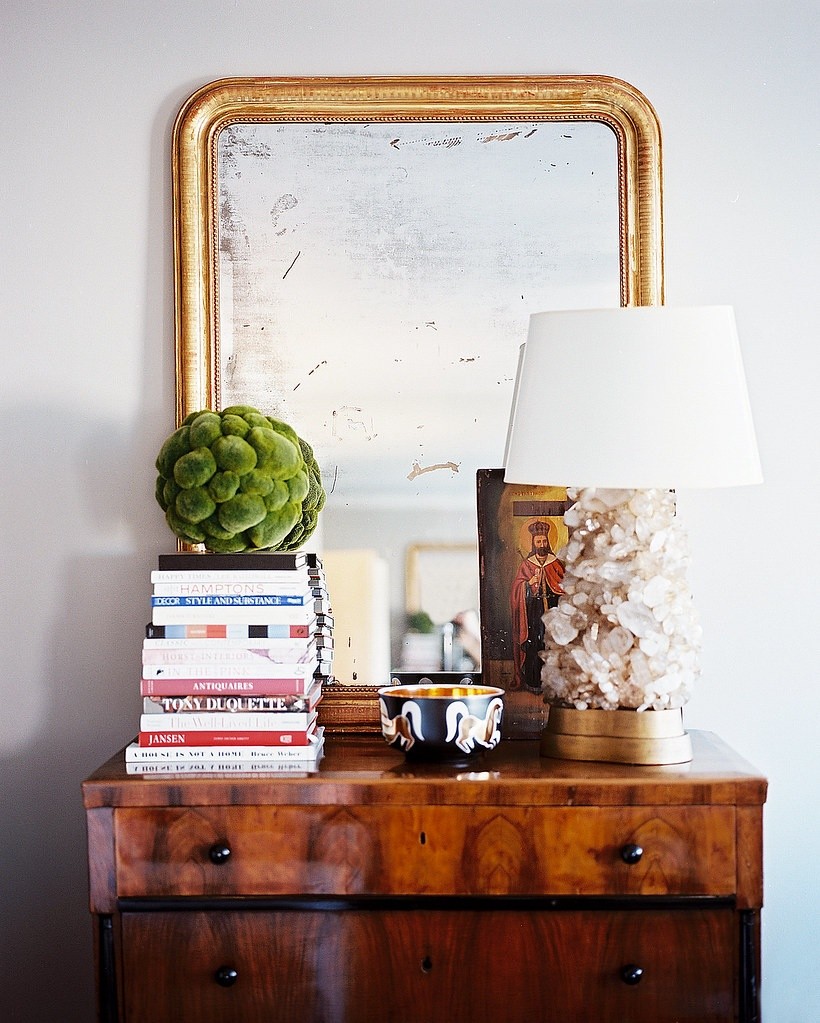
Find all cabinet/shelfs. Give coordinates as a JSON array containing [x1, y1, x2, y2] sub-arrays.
[[83, 726, 769, 1023]]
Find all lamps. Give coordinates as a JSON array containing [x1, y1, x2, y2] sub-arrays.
[[503, 303, 758, 765]]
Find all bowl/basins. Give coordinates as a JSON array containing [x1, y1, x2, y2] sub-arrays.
[[377, 684, 505, 769]]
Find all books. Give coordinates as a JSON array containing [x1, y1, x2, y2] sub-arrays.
[[124, 551, 326, 778], [307, 554, 335, 686]]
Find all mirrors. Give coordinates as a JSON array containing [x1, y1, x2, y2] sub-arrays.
[[174, 74, 666, 734]]
